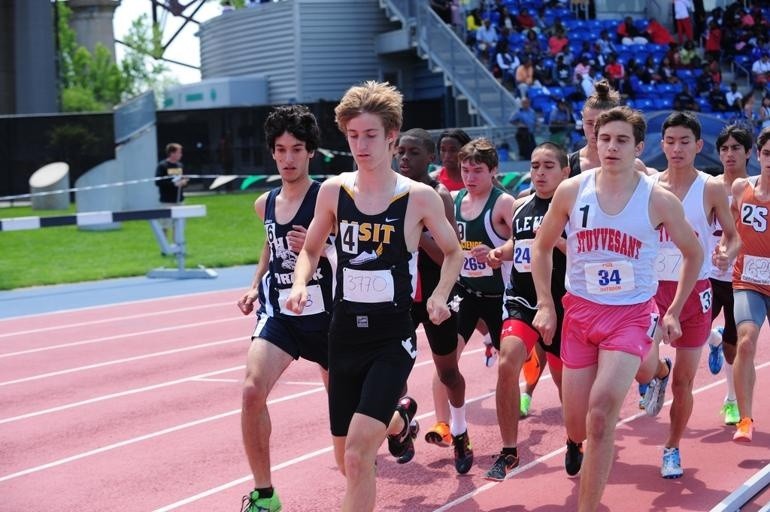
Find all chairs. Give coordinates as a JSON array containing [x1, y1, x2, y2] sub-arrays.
[[465, 0, 770, 153]]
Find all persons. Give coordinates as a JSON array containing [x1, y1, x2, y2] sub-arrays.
[[220, 0, 237, 17], [396, 127, 569, 482], [153, 143, 192, 256], [531, 106, 705, 512], [238, 105, 379, 512], [560, 80, 770, 512], [430, 1, 770, 162], [283, 83, 467, 512]]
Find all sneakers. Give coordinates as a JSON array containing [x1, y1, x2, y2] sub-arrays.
[[484, 452, 519, 481], [388, 397, 473, 473], [709, 328, 722, 374], [520, 392, 531, 417], [639, 358, 671, 416], [660, 446, 683, 478], [566, 439, 583, 476], [720, 398, 753, 441], [484, 340, 496, 367], [245, 488, 281, 512]]
